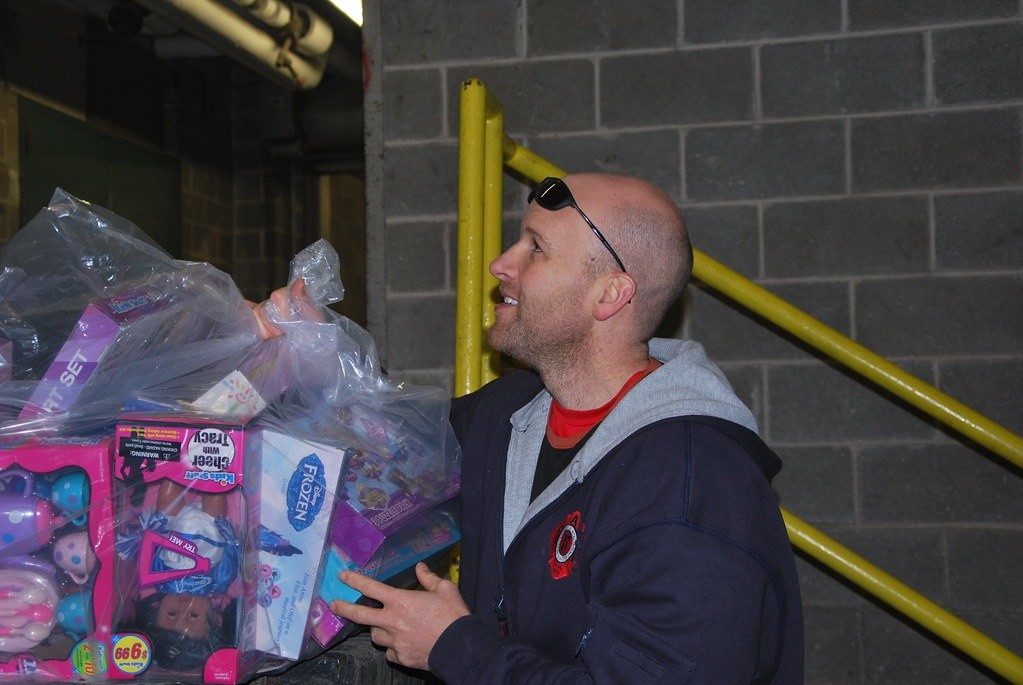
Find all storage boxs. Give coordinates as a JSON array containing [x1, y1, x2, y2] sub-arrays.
[[0, 265, 463, 685]]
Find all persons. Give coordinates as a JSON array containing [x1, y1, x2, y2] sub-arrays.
[[243, 171, 806, 685], [132, 478, 236, 672]]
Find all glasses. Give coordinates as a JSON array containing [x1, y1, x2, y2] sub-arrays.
[[527, 177, 631, 304]]
